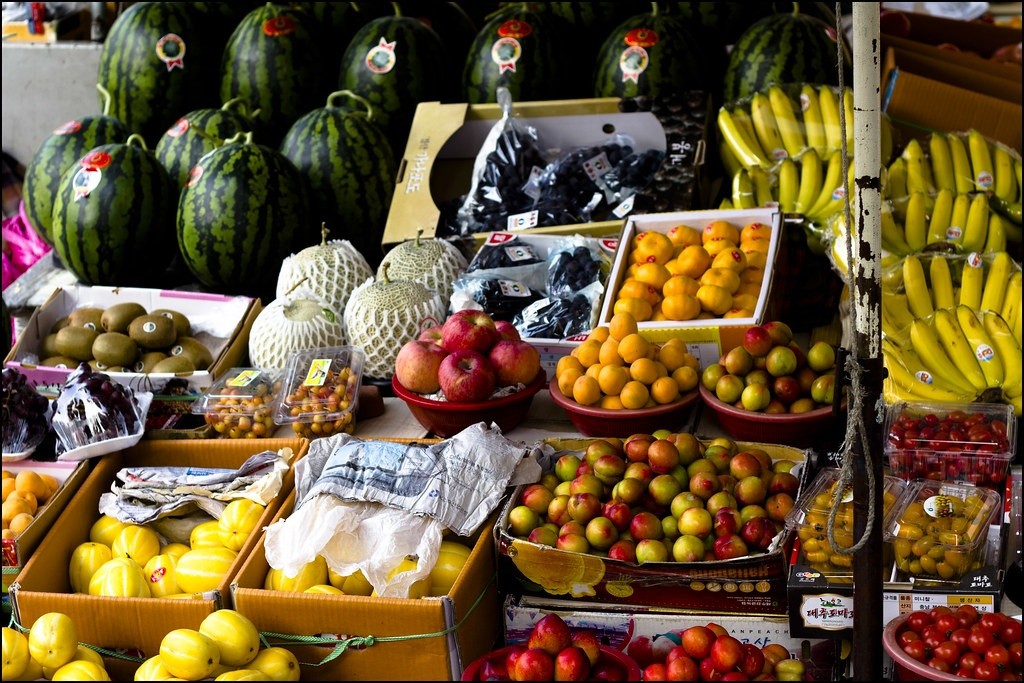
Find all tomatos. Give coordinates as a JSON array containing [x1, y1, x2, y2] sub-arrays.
[[898, 605, 1023, 680], [800, 480, 896, 583], [888, 410, 1009, 522], [893, 495, 989, 586]]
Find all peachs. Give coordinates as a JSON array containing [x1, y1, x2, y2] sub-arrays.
[[2, 470, 58, 539], [557, 312, 700, 409]]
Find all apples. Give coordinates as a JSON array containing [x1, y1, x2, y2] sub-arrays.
[[395, 309, 540, 402], [881, 9, 1024, 65]]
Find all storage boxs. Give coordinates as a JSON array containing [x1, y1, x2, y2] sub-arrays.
[[497, 589, 898, 683], [2, 278, 266, 398], [2, 452, 93, 592], [456, 218, 618, 379], [272, 347, 370, 436], [880, 394, 1019, 484], [1003, 464, 1022, 609], [782, 570, 1007, 643], [3, 40, 104, 168], [496, 425, 810, 606], [891, 482, 1006, 580], [880, 6, 1022, 81], [790, 460, 900, 568], [599, 203, 789, 369], [6, 431, 312, 679], [194, 359, 286, 434], [232, 429, 536, 681], [377, 97, 715, 248], [879, 45, 1022, 151]]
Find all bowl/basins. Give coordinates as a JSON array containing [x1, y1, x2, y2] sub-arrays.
[[883, 400, 1016, 483], [784, 467, 906, 567], [883, 479, 1001, 578], [392, 374, 546, 439], [274, 346, 364, 438], [549, 376, 699, 438], [191, 368, 281, 439], [882, 607, 987, 683], [699, 383, 847, 448]]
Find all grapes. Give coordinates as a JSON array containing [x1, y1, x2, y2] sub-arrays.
[[3, 362, 141, 455]]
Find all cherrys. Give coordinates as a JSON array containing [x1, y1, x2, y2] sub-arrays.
[[205, 367, 357, 438]]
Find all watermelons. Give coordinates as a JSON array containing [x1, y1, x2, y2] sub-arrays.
[[22, 0, 853, 291]]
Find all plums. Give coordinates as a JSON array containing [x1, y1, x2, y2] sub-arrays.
[[478, 612, 822, 682], [508, 429, 798, 563], [702, 322, 836, 414]]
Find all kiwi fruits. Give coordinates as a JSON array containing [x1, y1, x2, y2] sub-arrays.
[[41, 303, 213, 373]]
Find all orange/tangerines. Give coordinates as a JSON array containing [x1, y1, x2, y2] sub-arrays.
[[613, 221, 772, 321]]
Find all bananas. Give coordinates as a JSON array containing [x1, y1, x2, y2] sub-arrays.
[[717, 82, 1021, 419]]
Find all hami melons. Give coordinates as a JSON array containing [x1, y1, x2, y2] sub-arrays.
[[249, 222, 466, 378]]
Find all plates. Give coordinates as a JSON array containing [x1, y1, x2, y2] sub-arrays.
[[0, 399, 55, 462], [54, 390, 154, 460]]
